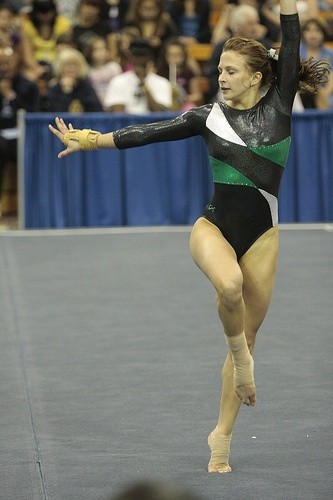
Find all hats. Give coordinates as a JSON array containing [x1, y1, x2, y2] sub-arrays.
[[129, 41, 154, 60]]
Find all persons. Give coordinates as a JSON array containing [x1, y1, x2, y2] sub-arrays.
[[0, 0, 332, 221], [49, 1, 331, 473]]
